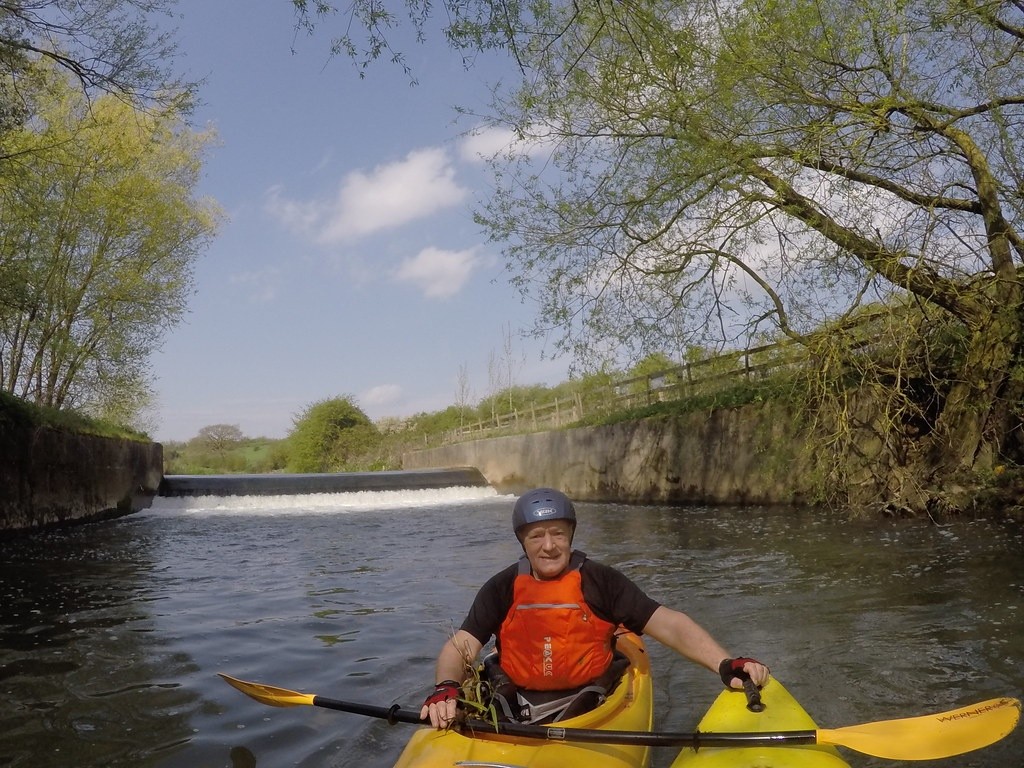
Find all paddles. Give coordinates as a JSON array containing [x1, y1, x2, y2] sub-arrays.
[[216, 668, 1024, 763]]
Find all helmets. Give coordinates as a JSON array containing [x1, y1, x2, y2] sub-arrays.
[[512, 488, 577, 532]]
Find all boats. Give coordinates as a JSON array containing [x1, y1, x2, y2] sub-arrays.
[[390, 627, 857, 768]]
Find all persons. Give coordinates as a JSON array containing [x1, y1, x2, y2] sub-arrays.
[[420, 488, 770, 728]]
